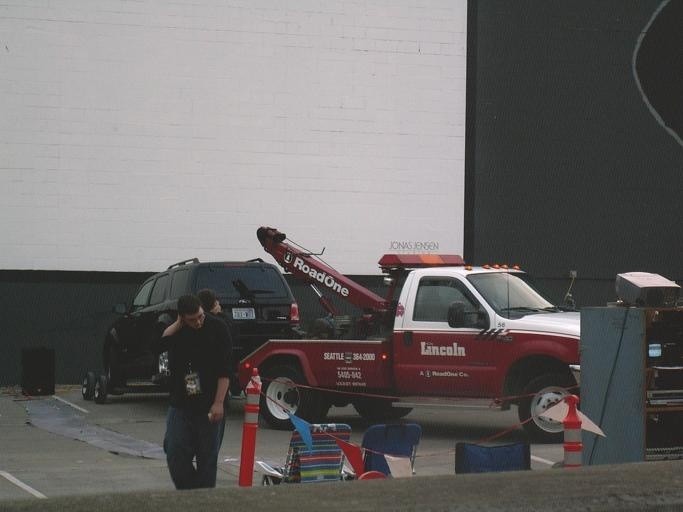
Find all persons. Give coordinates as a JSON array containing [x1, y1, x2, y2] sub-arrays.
[[196, 288, 234, 339], [155, 293, 234, 490]]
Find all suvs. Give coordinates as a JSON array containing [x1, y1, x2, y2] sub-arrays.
[[102, 256, 300, 390]]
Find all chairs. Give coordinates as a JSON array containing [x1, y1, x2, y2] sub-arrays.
[[453, 442, 533, 474], [256, 423, 420, 484]]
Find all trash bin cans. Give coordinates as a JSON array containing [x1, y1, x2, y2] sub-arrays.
[[21, 348, 55, 396]]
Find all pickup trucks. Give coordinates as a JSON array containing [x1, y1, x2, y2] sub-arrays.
[[237, 227, 581, 441]]
